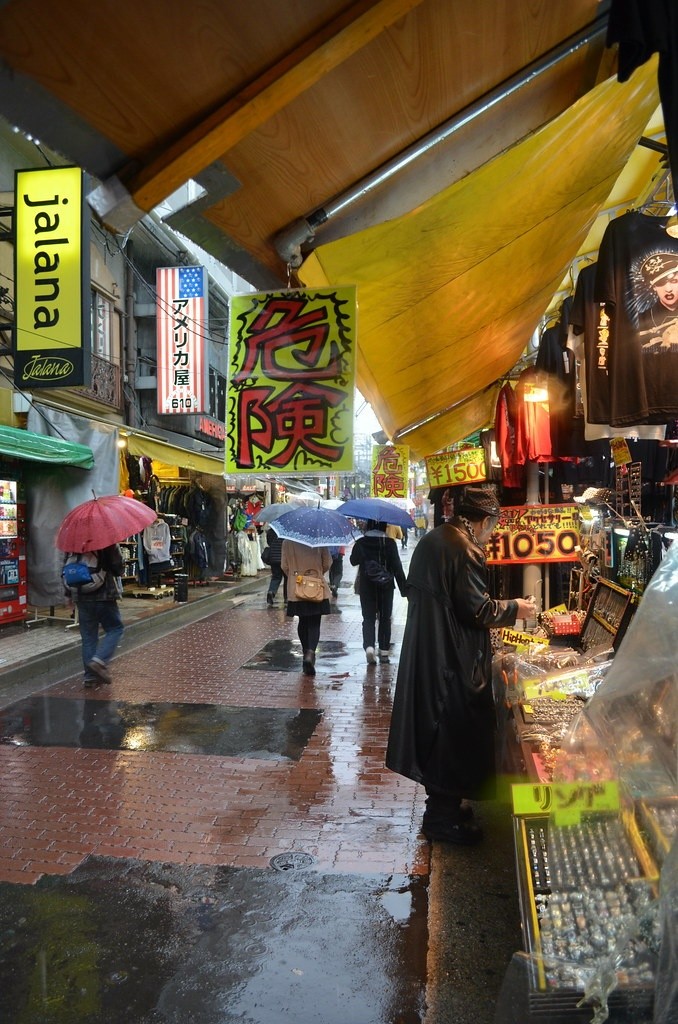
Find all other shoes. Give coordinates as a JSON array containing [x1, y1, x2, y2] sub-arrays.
[[423, 805, 473, 822], [366, 646, 377, 664], [267, 590, 273, 604], [422, 821, 484, 848], [302, 649, 315, 675], [86, 660, 112, 684], [85, 680, 103, 687], [329, 585, 337, 598], [375, 647, 389, 657], [284, 599, 287, 603]]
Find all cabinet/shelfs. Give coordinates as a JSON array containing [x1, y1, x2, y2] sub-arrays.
[[118, 525, 184, 582]]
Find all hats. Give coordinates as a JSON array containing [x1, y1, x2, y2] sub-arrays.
[[462, 488, 503, 517], [120, 489, 143, 500], [573, 487, 617, 505]]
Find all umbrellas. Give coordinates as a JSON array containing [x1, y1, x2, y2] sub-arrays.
[[289, 492, 345, 510], [254, 502, 296, 523], [375, 497, 417, 510], [336, 497, 417, 528], [56, 489, 158, 554], [270, 499, 365, 548]]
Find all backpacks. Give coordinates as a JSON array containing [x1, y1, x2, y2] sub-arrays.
[[61, 549, 111, 593], [233, 508, 252, 531], [328, 545, 341, 560]]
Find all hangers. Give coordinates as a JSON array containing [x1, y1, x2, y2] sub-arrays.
[[636, 173, 676, 216]]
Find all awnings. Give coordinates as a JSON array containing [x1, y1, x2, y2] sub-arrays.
[[0, 0, 658, 464], [0, 421, 95, 471]]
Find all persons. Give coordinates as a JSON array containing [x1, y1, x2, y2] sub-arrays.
[[386, 485, 536, 838], [142, 519, 174, 586], [267, 513, 428, 676], [61, 543, 124, 687]]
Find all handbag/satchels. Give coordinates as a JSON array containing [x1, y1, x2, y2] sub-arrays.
[[261, 547, 271, 562], [363, 560, 394, 586], [295, 569, 324, 602]]
